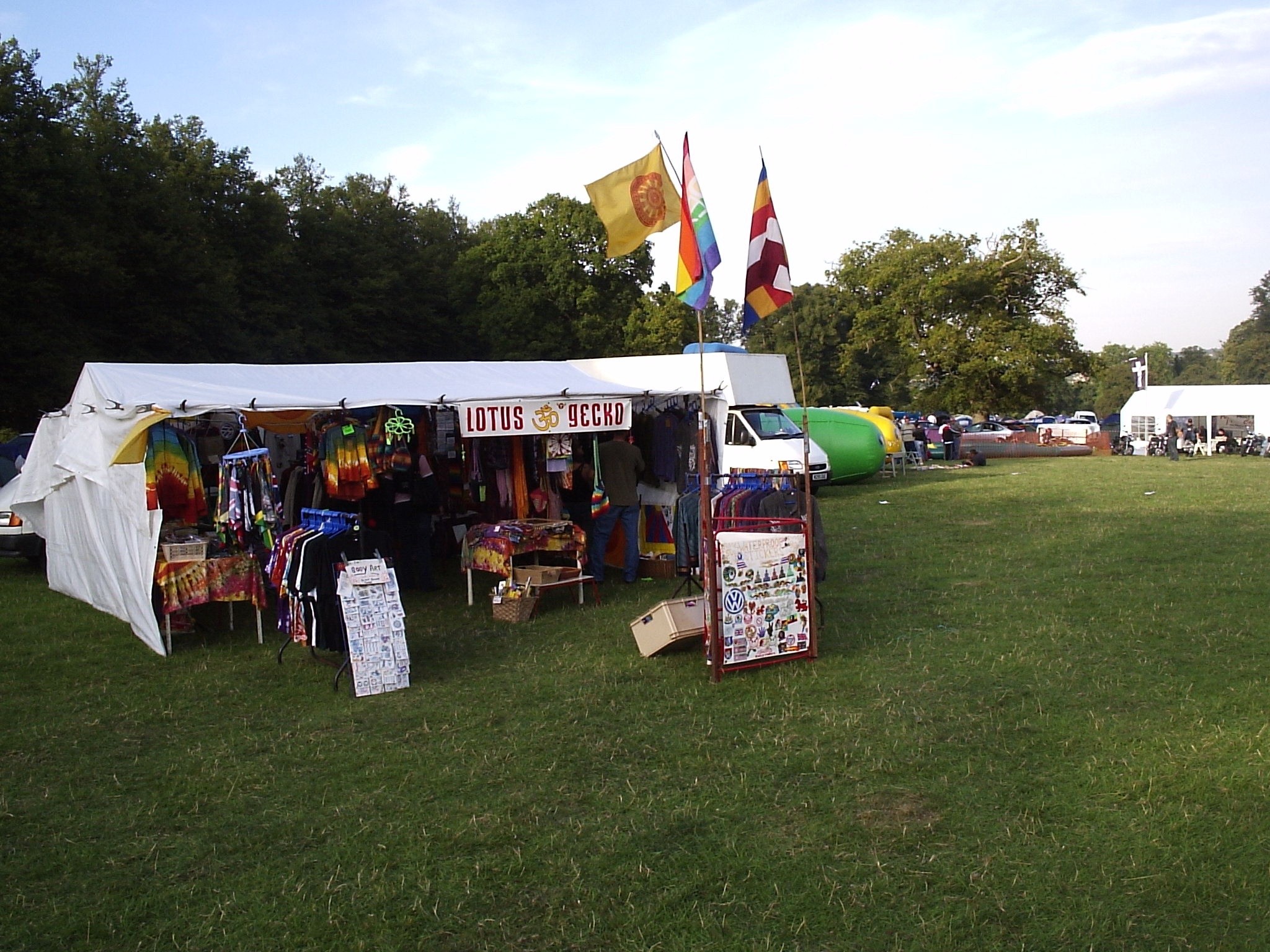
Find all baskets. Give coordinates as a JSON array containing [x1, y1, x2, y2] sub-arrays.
[[490, 576, 539, 621], [160, 540, 210, 564]]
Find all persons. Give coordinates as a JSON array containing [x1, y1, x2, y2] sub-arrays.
[[901, 417, 928, 465], [938, 419, 962, 461], [587, 429, 646, 584], [1165, 415, 1180, 461], [962, 449, 986, 466], [393, 432, 443, 591], [1181, 418, 1207, 457], [1215, 429, 1229, 454]]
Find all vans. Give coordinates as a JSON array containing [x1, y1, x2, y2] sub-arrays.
[[1074, 411, 1100, 433]]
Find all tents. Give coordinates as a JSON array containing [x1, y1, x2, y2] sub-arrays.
[[11, 354, 796, 658]]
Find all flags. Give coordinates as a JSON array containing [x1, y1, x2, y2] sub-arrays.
[[584, 143, 681, 258], [742, 166, 794, 330], [675, 132, 721, 312]]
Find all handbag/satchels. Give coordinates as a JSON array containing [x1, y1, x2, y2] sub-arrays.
[[591, 483, 611, 521]]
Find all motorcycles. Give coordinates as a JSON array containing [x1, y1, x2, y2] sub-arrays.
[[1109, 430, 1137, 456], [1240, 426, 1265, 457]]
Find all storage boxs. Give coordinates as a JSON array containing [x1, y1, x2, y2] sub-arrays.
[[630, 592, 705, 657], [159, 535, 207, 562], [513, 564, 563, 584]]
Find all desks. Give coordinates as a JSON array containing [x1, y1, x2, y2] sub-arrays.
[[156, 549, 267, 656], [464, 530, 585, 606]]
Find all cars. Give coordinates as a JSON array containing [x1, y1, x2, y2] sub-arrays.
[[891, 410, 1091, 444], [0, 432, 48, 573]]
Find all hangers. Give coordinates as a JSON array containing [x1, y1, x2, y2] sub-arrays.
[[296, 506, 358, 541], [641, 395, 700, 421], [682, 473, 798, 505]]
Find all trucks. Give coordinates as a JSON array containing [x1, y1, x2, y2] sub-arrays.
[[564, 352, 831, 498]]
